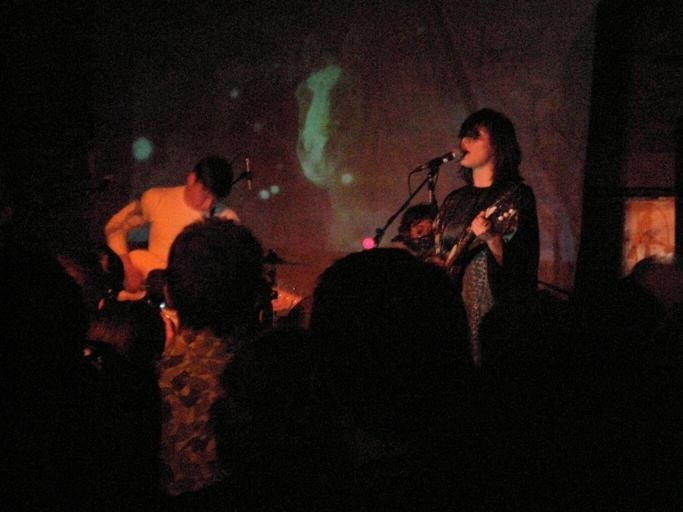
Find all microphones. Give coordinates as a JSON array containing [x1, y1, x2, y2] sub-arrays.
[[242, 159, 254, 190], [413, 149, 463, 170]]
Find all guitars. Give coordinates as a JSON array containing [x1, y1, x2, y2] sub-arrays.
[[117, 250, 168, 301], [443, 191, 517, 276]]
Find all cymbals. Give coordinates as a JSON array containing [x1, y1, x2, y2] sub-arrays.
[[263, 255, 309, 268]]
